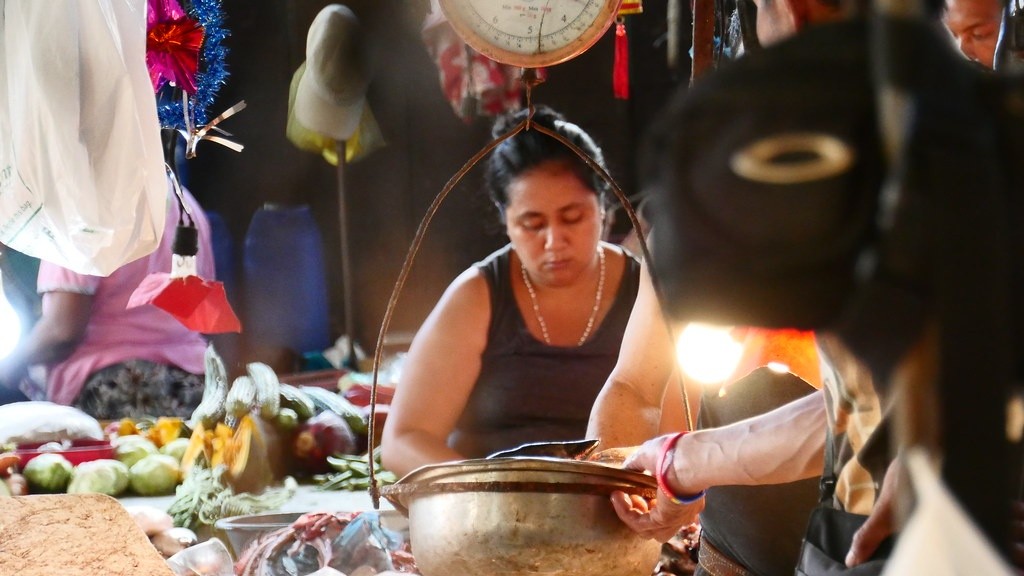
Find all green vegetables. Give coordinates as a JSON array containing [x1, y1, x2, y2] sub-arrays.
[[166, 463, 295, 529]]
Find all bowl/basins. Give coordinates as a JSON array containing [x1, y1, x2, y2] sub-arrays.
[[215, 513, 318, 561], [15, 441, 113, 471], [385, 453, 670, 576]]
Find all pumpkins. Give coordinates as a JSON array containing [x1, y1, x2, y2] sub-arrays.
[[117, 417, 280, 489]]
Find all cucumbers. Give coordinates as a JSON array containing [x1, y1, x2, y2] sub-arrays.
[[193, 344, 370, 432]]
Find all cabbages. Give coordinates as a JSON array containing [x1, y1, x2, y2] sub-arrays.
[[24, 433, 189, 500]]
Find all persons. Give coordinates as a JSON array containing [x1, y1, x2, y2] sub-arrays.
[[611, 0, 964, 576], [0, 176, 215, 423], [379, 105, 674, 481], [943, 0, 1002, 70]]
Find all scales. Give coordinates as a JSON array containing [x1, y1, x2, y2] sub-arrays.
[[367, 0, 693, 576]]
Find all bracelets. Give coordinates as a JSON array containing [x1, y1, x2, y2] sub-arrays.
[[656, 430, 706, 503]]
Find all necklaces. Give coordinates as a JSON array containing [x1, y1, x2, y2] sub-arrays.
[[521, 246, 606, 346]]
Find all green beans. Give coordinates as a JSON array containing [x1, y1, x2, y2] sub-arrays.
[[314, 446, 395, 491]]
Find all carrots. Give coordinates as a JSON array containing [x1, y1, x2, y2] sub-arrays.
[[0, 453, 27, 496]]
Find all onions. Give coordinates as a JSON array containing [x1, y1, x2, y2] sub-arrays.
[[292, 409, 355, 474]]
